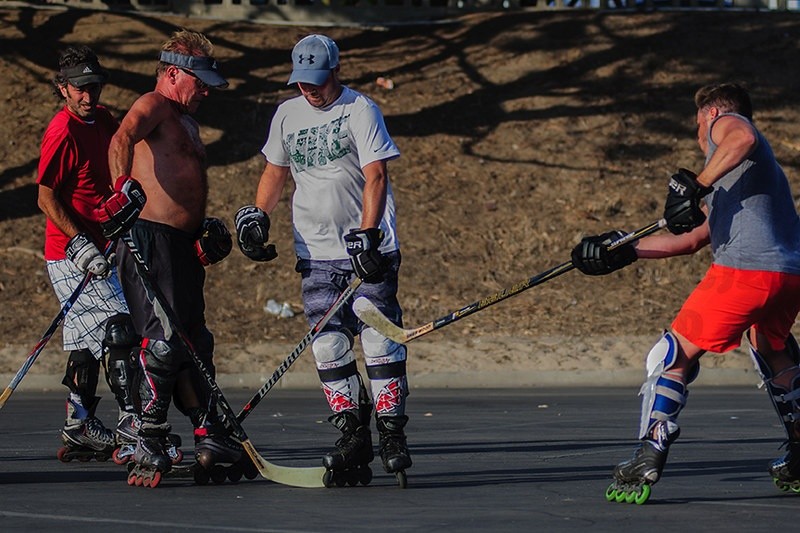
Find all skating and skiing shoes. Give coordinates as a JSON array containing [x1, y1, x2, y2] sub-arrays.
[[605, 419, 683, 504], [113, 406, 183, 463], [188, 410, 259, 487], [57, 392, 119, 461], [767, 437, 800, 492], [127, 420, 171, 488], [323, 403, 378, 486], [376, 413, 414, 487]]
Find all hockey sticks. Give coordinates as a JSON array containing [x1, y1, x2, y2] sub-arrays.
[[0, 240, 115, 409], [122, 232, 327, 490], [353, 219, 668, 346], [236, 276, 363, 422]]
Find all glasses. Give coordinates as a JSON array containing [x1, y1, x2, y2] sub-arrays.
[[175, 66, 214, 89]]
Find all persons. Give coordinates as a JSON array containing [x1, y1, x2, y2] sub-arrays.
[[35, 28, 259, 474], [572, 82, 800, 487], [235, 36, 412, 473]]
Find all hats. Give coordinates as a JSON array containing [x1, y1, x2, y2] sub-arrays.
[[287, 34, 338, 85], [57, 64, 102, 87], [161, 51, 229, 90]]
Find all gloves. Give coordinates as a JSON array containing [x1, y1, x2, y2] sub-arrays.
[[572, 230, 639, 276], [94, 175, 146, 240], [64, 232, 117, 280], [663, 168, 714, 236], [192, 217, 233, 268], [344, 228, 392, 283], [234, 205, 278, 262]]
